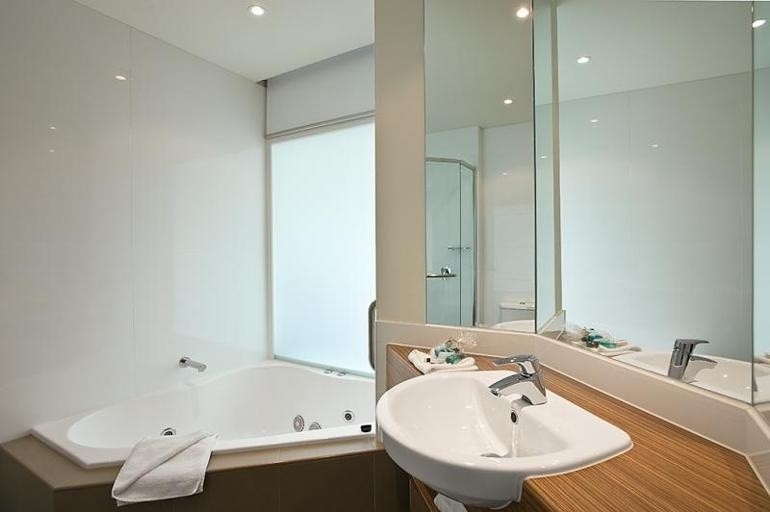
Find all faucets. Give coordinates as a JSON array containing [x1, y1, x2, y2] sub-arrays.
[[179, 356, 207, 373], [487, 354, 547, 404], [666, 338, 718, 380]]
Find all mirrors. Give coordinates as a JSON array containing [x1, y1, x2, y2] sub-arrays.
[[534, 1, 752, 403], [424, 0, 536, 335], [751, 1, 770, 428]]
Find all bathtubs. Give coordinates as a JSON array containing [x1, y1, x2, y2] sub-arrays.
[[29, 358, 376, 472]]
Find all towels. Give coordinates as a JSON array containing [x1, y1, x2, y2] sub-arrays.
[[408, 348, 479, 376], [111, 433, 212, 506], [569, 336, 640, 359]]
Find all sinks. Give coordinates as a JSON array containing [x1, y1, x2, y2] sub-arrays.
[[609, 351, 770, 406], [375, 369, 635, 510], [489, 320, 545, 333]]
